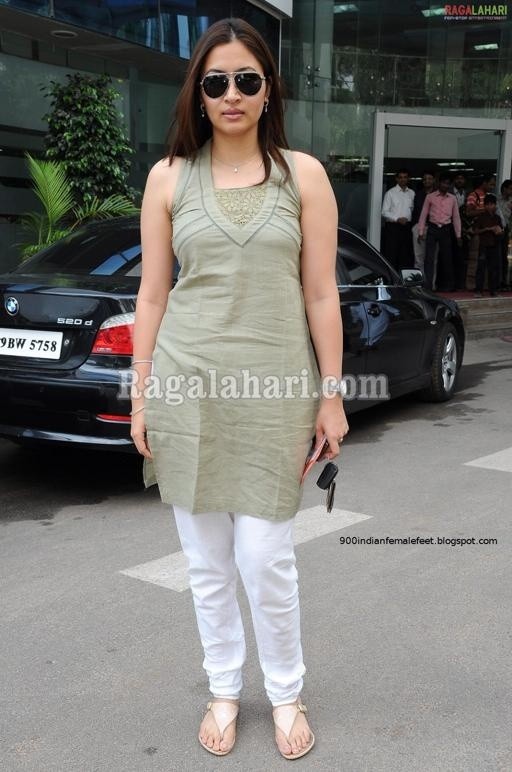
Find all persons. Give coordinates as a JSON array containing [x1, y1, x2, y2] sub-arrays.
[[379, 170, 512, 297], [127, 16, 350, 760]]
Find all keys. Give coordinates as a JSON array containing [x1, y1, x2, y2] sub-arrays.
[[316, 463, 338, 490]]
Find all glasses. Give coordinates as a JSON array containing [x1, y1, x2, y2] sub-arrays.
[[200, 71, 267, 99]]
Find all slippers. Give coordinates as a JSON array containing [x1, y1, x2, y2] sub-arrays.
[[272, 696, 315, 759], [198, 698, 239, 755]]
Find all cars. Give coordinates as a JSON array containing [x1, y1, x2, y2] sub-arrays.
[[1, 214, 465, 457]]
